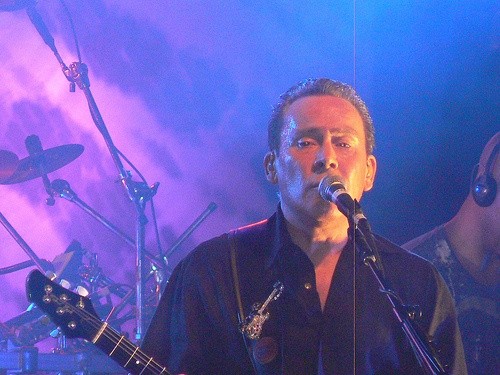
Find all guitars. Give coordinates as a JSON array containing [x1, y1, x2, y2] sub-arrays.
[[25, 268, 173, 375]]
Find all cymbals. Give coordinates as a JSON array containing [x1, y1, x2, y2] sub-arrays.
[[0, 143, 84, 185]]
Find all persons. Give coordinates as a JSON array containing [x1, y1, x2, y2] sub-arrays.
[[140, 78, 468, 375], [400, 130, 500, 375]]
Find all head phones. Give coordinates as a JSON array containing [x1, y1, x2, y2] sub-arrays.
[[472, 144, 500, 208]]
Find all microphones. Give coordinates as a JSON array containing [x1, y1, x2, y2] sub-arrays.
[[317, 175, 379, 260]]
